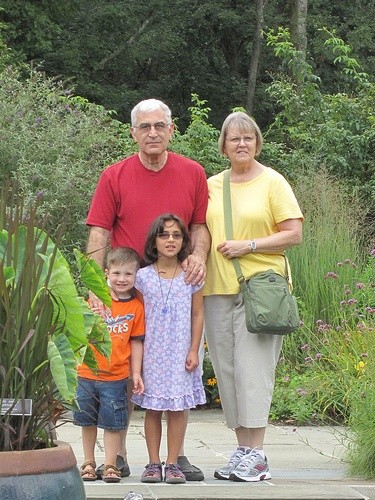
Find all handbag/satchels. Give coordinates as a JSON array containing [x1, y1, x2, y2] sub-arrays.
[[242, 269, 299, 335]]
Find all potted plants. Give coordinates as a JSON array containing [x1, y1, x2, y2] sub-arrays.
[[0, 166, 106, 500]]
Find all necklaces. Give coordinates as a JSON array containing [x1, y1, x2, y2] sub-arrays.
[[157, 259, 180, 315]]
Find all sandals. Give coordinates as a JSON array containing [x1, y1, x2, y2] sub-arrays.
[[102, 465, 122, 481], [80, 460, 96, 480]]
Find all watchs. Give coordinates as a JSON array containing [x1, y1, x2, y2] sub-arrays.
[[250, 239, 256, 253]]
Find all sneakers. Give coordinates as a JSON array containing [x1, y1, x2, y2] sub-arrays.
[[142, 463, 164, 482], [214, 447, 247, 480], [229, 446, 272, 481], [166, 463, 186, 482]]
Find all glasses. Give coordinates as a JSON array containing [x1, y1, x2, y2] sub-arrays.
[[133, 122, 170, 132], [157, 230, 183, 240]]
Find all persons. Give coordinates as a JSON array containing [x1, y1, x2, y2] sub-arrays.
[[87, 98, 211, 481], [73, 248, 145, 484], [130, 213, 204, 484], [206, 112, 305, 482]]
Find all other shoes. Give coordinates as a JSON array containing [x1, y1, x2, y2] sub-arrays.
[[95, 455, 130, 476], [177, 455, 205, 480]]
